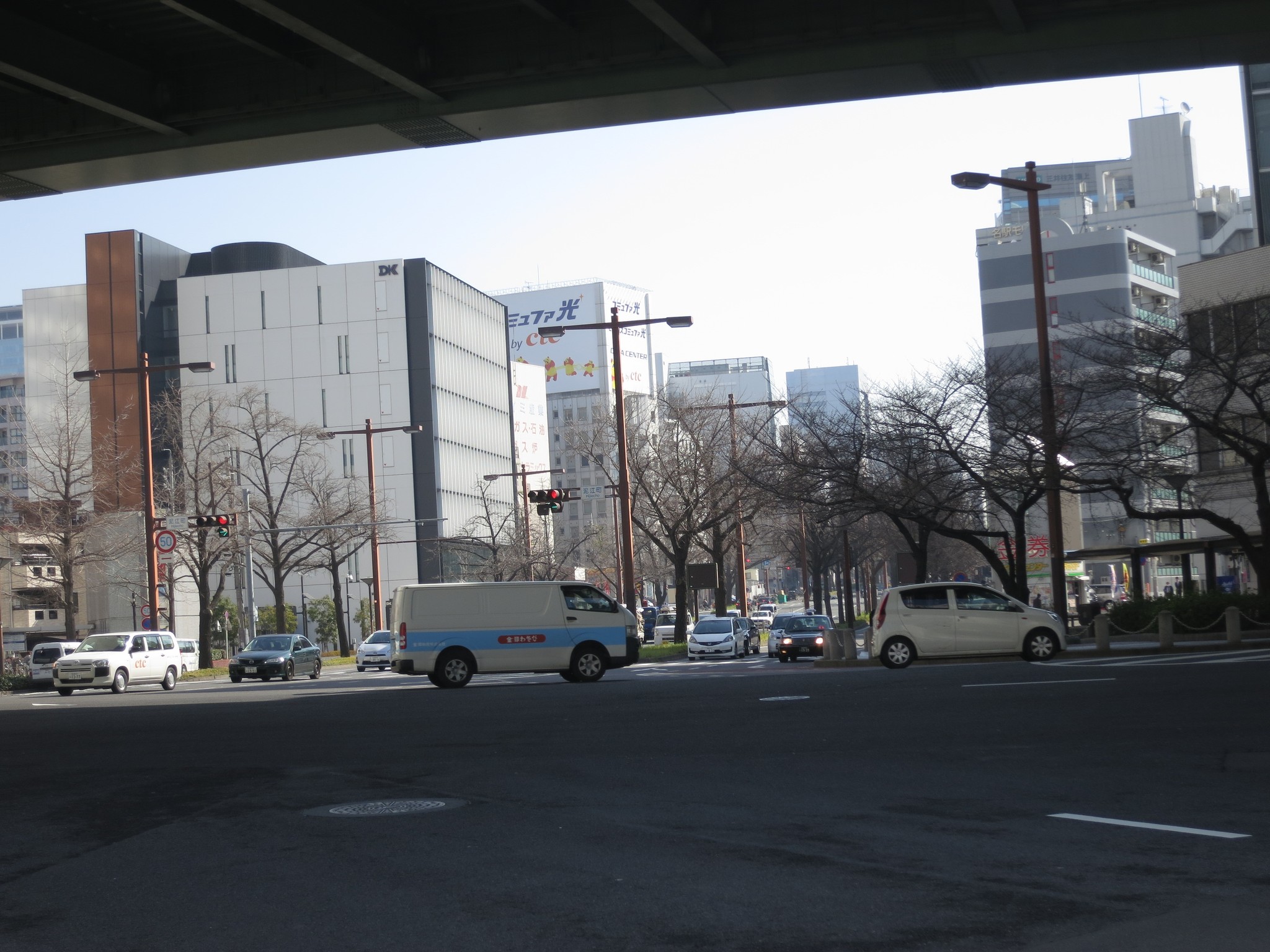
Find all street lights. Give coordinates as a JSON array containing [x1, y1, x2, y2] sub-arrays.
[[360, 576, 374, 634], [951, 159, 1073, 631], [670, 391, 792, 617], [73, 352, 215, 631], [539, 307, 693, 617], [316, 418, 424, 630], [484, 464, 565, 581], [1161, 471, 1194, 598]]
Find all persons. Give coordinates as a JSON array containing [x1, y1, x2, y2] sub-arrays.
[[700, 599, 708, 610], [1175, 577, 1182, 598], [563, 590, 576, 609], [1162, 582, 1174, 597], [661, 616, 671, 625], [1033, 593, 1042, 609]]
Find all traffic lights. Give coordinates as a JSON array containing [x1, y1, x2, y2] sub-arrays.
[[548, 501, 564, 513], [216, 526, 230, 538], [195, 514, 239, 527], [527, 488, 566, 503]]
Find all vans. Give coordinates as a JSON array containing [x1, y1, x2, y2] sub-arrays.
[[29, 642, 94, 688], [52, 632, 183, 696], [165, 639, 200, 674], [387, 580, 641, 689]]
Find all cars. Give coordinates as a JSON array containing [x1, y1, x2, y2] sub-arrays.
[[638, 592, 838, 662], [355, 630, 391, 673], [228, 633, 323, 683], [869, 581, 1068, 670], [1090, 584, 1128, 612]]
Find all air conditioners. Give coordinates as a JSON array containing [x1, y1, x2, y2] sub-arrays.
[[1172, 554, 1182, 563], [1132, 286, 1141, 296], [1150, 254, 1163, 262], [1153, 295, 1165, 306], [1161, 424, 1176, 433], [1128, 242, 1137, 254]]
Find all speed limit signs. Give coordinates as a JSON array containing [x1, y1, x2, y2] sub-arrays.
[[139, 604, 151, 617], [155, 531, 177, 554]]
